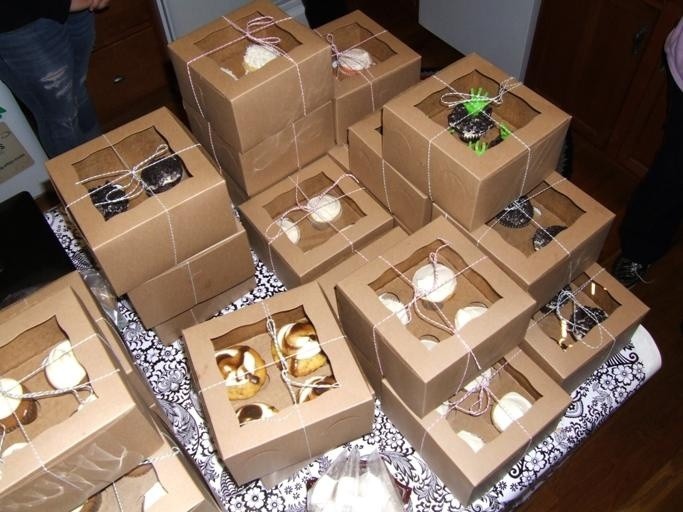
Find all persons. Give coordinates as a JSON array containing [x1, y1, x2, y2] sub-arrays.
[[0, 0, 108, 156], [610, 15, 682, 289]]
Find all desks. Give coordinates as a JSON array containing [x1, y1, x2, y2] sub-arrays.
[[44, 203, 666, 512]]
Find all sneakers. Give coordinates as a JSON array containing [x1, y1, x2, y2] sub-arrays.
[[611, 254, 651, 293]]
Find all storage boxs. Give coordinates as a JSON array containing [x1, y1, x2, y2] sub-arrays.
[[375, 48, 575, 234], [1, 271, 154, 412], [0, 285, 165, 511], [164, 3, 336, 154], [176, 102, 336, 199], [41, 104, 239, 303], [510, 261, 649, 398], [153, 275, 260, 348], [333, 213, 540, 414], [53, 406, 225, 512], [235, 155, 391, 285], [175, 280, 375, 487], [375, 344, 573, 507], [122, 220, 258, 329], [343, 111, 436, 235], [428, 167, 619, 314], [292, 7, 425, 135]]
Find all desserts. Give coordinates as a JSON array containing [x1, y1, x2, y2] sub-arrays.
[[0, 44, 608, 511]]
